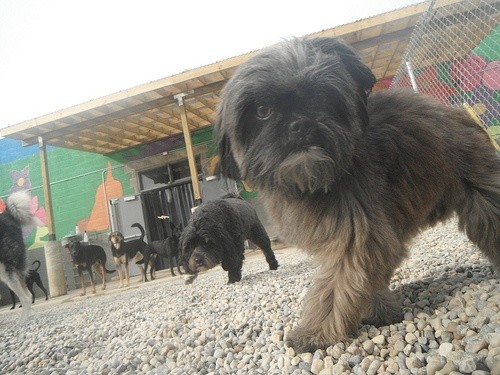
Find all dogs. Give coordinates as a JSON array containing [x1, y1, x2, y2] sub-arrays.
[[61, 222, 183, 296], [178, 191, 280, 285], [0, 191, 48, 326], [213, 35, 500, 354]]
